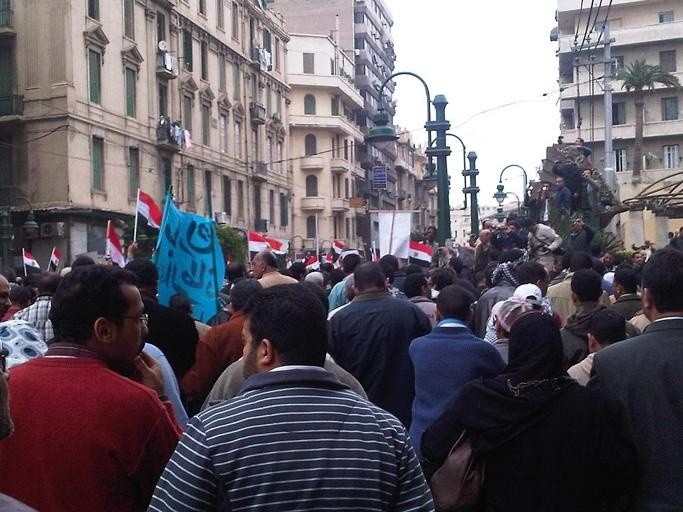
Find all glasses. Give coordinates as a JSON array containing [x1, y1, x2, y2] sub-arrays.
[[110, 314, 149, 323]]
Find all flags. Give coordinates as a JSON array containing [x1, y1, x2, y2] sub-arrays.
[[51, 246, 62, 271], [303, 257, 319, 271], [107, 222, 126, 268], [322, 255, 333, 265], [248, 230, 283, 253], [22, 248, 42, 273], [139, 190, 163, 228], [332, 240, 344, 254], [407, 239, 434, 268]]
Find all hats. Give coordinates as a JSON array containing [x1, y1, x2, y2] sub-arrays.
[[513, 282, 541, 304]]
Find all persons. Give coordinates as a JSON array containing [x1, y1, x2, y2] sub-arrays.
[[550, 177, 572, 218], [418, 227, 439, 253], [450, 256, 477, 294], [556, 268, 631, 369], [164, 293, 212, 380], [632, 240, 655, 262], [318, 262, 334, 281], [378, 264, 408, 301], [475, 250, 529, 336], [568, 308, 637, 387], [506, 220, 528, 249], [124, 261, 197, 381], [588, 244, 602, 266], [0, 264, 183, 512], [321, 262, 433, 428], [549, 252, 574, 284], [425, 268, 456, 303], [378, 254, 408, 294], [325, 269, 348, 297], [668, 226, 683, 248], [523, 220, 563, 271], [403, 265, 422, 275], [285, 261, 307, 284], [0, 275, 13, 320], [218, 262, 245, 296], [0, 340, 38, 512], [14, 272, 66, 346], [608, 261, 642, 304], [474, 230, 499, 264], [585, 245, 683, 511], [407, 285, 507, 442], [305, 273, 325, 291], [139, 343, 191, 429], [429, 246, 450, 271], [667, 232, 673, 240], [511, 282, 543, 308], [580, 169, 603, 231], [599, 253, 617, 274], [481, 219, 492, 233], [327, 253, 362, 312], [605, 264, 645, 320], [639, 250, 648, 263], [566, 218, 596, 256], [546, 251, 610, 323], [487, 299, 532, 367], [402, 274, 439, 329], [418, 310, 634, 512], [552, 157, 582, 214], [467, 234, 478, 246], [144, 285, 434, 512], [250, 251, 299, 287], [200, 283, 369, 409], [629, 252, 644, 268], [491, 224, 506, 251], [630, 302, 656, 336], [178, 278, 263, 409], [2, 286, 34, 320], [571, 138, 593, 157], [549, 255, 564, 280], [482, 262, 550, 344], [535, 182, 556, 225]]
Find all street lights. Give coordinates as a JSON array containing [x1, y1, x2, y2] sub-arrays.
[[497, 191, 521, 216], [287, 235, 305, 254], [0, 198, 40, 268]]
